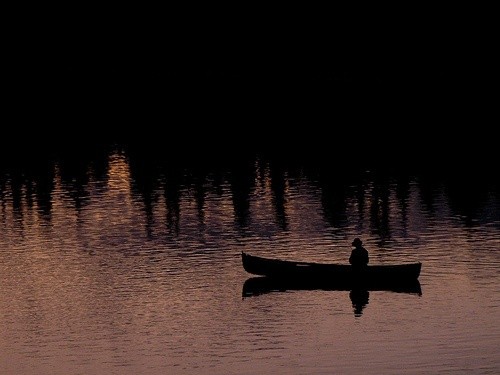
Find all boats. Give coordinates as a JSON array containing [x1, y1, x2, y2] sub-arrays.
[[241, 251, 422, 280], [241, 276, 422, 297]]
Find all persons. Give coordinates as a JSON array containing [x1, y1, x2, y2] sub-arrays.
[[348, 237, 369, 266]]
